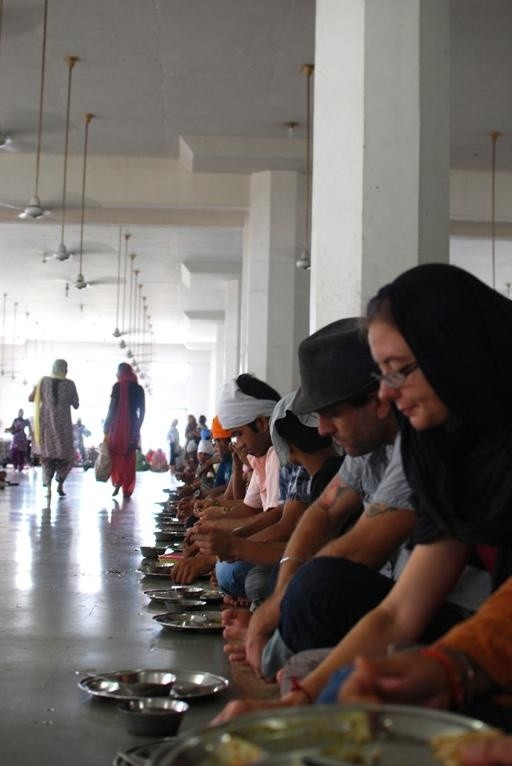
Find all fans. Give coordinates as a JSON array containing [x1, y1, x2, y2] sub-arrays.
[[0, 0, 115, 292], [283, 61, 313, 271], [109, 225, 152, 386]]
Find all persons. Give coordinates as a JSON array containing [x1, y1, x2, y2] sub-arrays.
[[3, 408, 33, 472], [28, 359, 79, 497], [26, 439, 168, 472], [102, 363, 146, 499], [169, 262, 511, 765], [70, 418, 93, 462]]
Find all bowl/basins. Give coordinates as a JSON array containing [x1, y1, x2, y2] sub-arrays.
[[118, 698, 189, 738], [115, 736, 175, 765]]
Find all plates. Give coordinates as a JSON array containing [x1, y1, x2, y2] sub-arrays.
[[77, 668, 229, 702], [158, 700, 511, 766], [137, 489, 226, 633]]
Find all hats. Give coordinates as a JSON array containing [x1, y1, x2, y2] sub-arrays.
[[291, 318, 378, 414]]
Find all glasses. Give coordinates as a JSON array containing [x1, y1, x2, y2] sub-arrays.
[[371, 361, 420, 388]]
